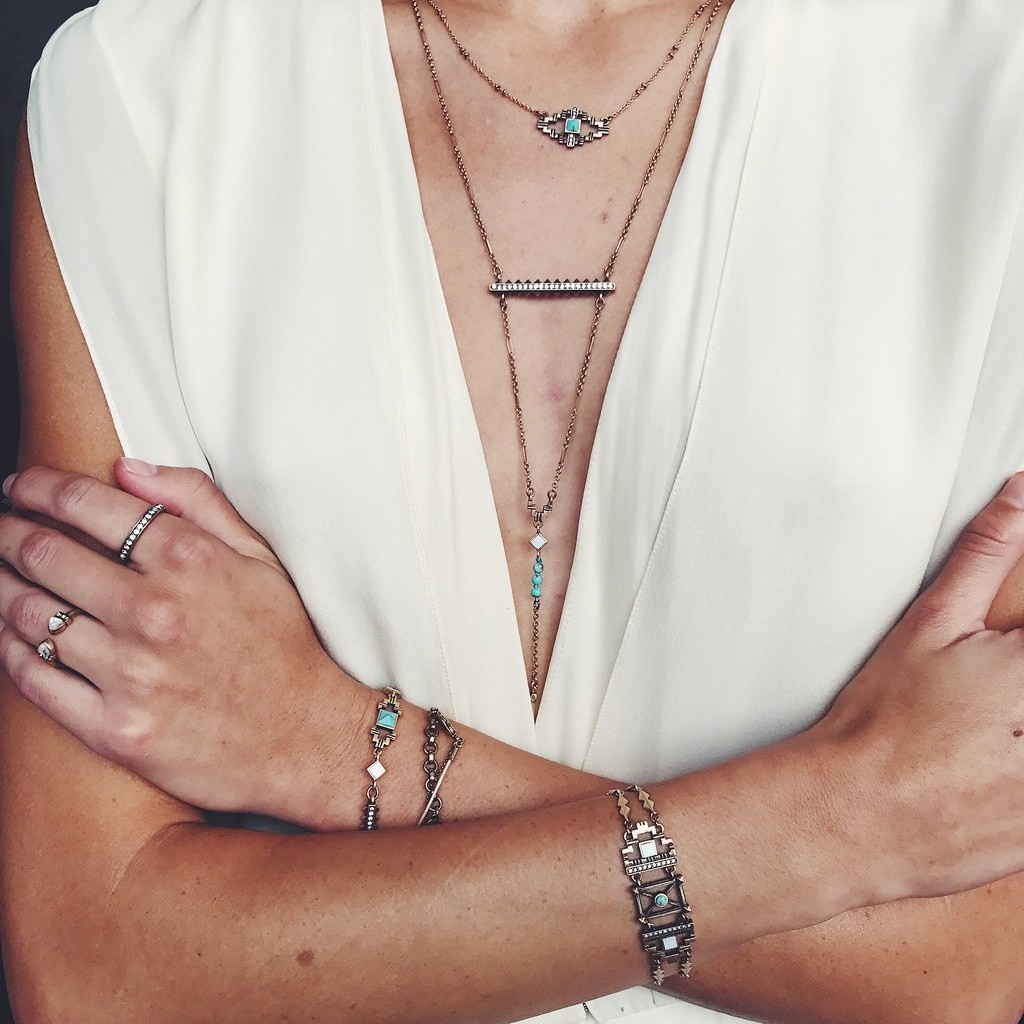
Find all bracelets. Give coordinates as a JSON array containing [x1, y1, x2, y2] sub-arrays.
[[605, 782, 694, 987], [416, 706, 464, 826], [348, 684, 411, 828]]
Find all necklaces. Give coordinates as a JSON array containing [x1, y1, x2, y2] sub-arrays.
[[412, 0, 725, 705]]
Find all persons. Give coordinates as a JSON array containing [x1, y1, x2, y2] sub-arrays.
[[4, 0, 1021, 1024]]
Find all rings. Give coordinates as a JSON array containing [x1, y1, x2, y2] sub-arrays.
[[35, 606, 86, 668], [116, 505, 166, 565]]
